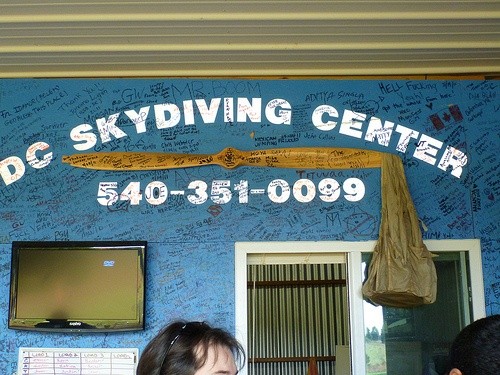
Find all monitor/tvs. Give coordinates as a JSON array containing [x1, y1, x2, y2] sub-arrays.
[[8, 240, 147, 332]]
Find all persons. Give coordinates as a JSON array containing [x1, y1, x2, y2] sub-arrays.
[[136, 319, 245, 375], [441, 314, 500, 375]]
